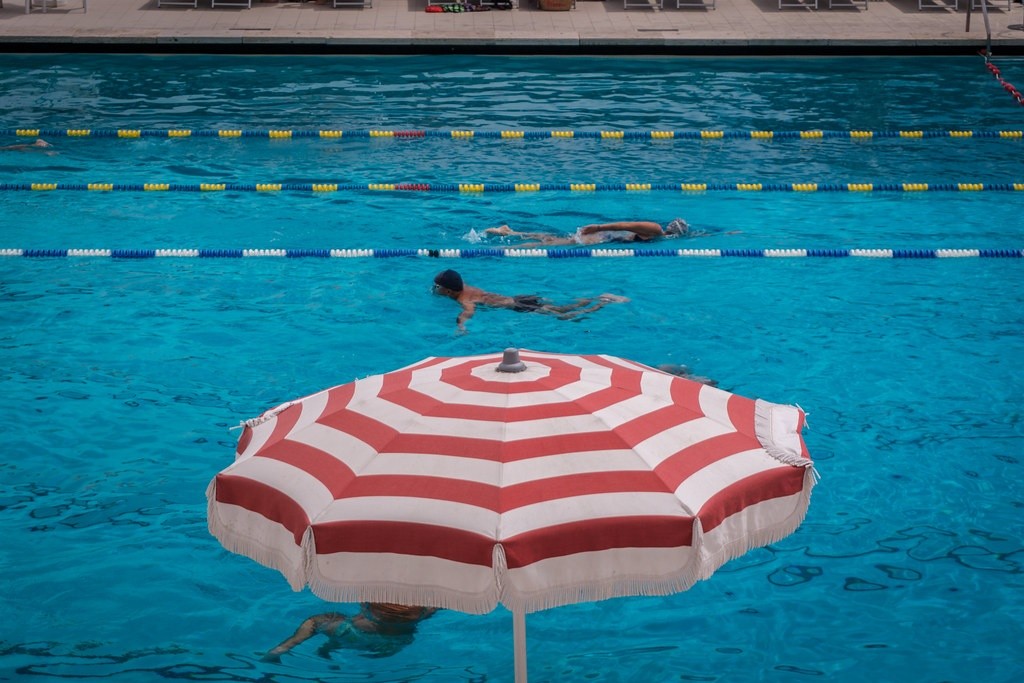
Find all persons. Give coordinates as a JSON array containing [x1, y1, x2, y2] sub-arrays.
[[485, 218, 691, 248], [431, 268, 629, 335], [257, 594, 440, 666]]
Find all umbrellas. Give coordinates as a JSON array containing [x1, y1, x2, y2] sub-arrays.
[[202, 347, 821, 682]]
